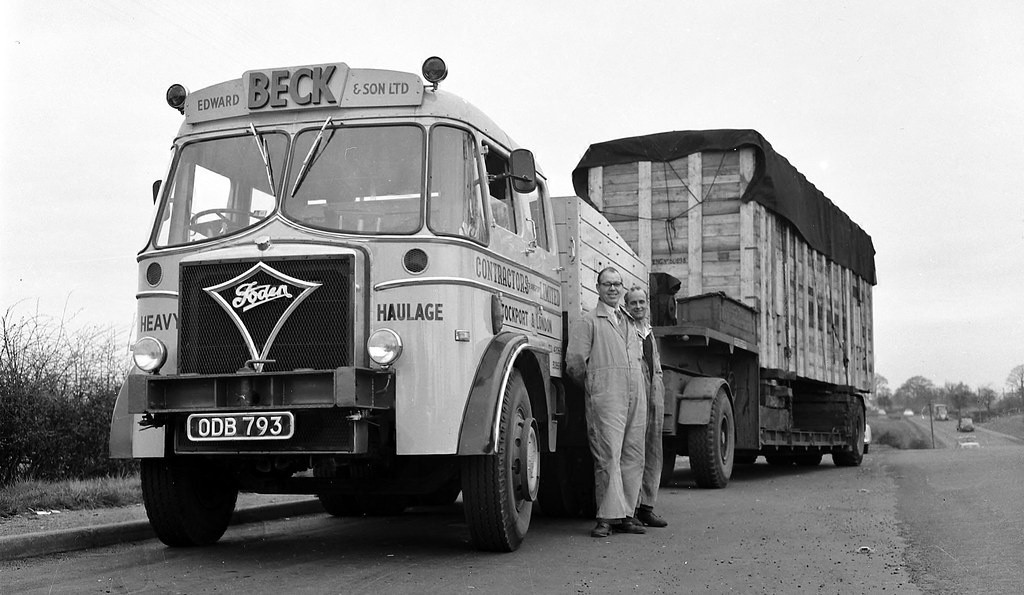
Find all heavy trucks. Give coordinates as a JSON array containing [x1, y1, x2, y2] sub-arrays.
[[109, 55, 878, 557]]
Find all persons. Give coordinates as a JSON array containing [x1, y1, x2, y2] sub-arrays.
[[566, 267, 648, 538], [624, 287, 668, 527]]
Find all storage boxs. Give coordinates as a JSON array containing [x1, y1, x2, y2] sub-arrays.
[[675, 292, 758, 346]]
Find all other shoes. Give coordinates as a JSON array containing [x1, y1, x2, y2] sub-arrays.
[[612, 517, 647, 534], [632, 517, 642, 526], [591, 521, 612, 537], [636, 508, 668, 527]]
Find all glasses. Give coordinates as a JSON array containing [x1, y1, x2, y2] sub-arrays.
[[598, 281, 623, 288]]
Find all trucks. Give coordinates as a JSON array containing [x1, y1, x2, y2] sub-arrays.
[[933, 403, 950, 421]]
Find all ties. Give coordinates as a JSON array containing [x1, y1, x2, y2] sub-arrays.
[[615, 310, 626, 335]]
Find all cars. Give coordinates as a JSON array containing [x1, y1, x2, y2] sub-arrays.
[[957, 417, 975, 432], [863, 422, 872, 453], [903, 409, 914, 418]]
